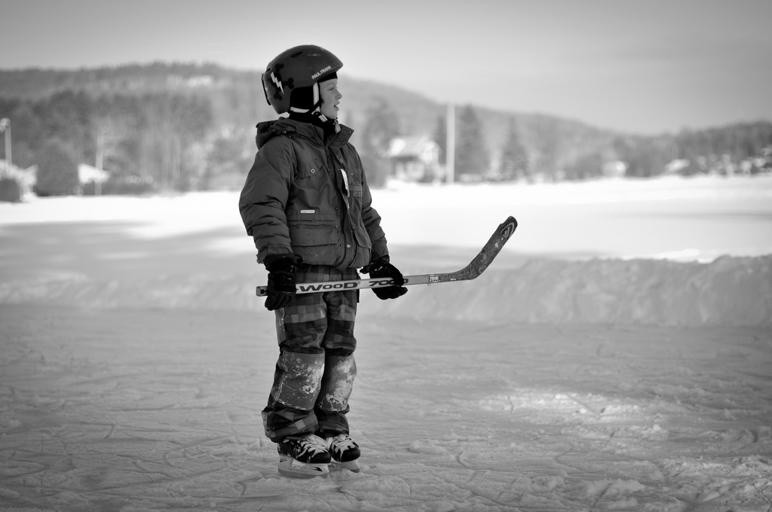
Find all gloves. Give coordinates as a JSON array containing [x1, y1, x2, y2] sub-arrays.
[[368, 259, 407, 300], [263, 253, 303, 310]]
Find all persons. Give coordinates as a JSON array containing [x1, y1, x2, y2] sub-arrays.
[[239, 46, 407, 463]]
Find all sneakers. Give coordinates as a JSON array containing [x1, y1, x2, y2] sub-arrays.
[[318, 429, 360, 462], [277, 432, 331, 463]]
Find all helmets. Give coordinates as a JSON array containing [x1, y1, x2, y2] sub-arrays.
[[261, 45, 343, 114]]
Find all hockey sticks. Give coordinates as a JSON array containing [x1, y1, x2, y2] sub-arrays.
[[255, 216, 517, 296]]
